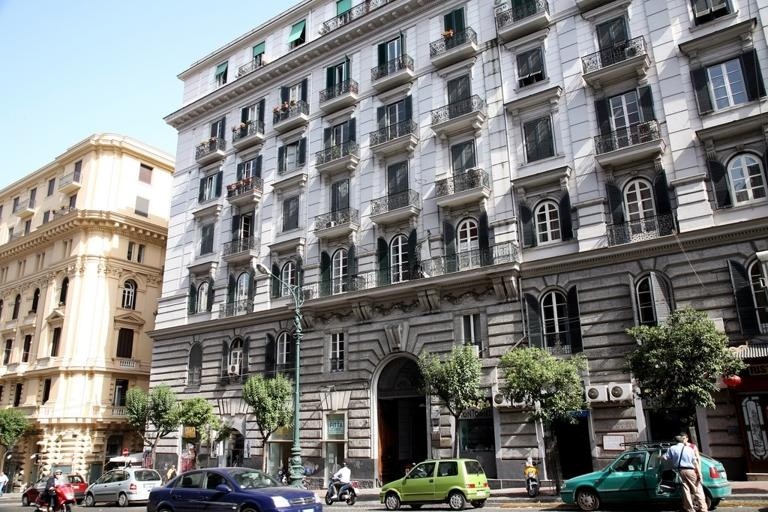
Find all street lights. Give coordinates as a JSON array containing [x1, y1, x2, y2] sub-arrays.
[[30, 452, 43, 481], [256, 264, 307, 490]]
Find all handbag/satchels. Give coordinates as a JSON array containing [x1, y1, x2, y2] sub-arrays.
[[671, 467, 683, 485]]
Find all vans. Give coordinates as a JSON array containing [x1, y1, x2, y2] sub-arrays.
[[84, 468, 163, 507]]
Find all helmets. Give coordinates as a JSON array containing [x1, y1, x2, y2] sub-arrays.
[[341, 462, 346, 467]]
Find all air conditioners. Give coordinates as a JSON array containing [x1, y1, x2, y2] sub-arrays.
[[494, 3, 509, 17], [636, 119, 658, 138], [460, 344, 480, 359], [624, 42, 643, 60], [465, 166, 482, 186], [492, 0, 508, 8], [706, 316, 726, 334], [228, 364, 240, 375], [491, 382, 635, 409]]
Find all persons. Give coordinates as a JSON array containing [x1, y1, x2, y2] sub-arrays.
[[43, 470, 67, 512], [0, 472, 9, 497], [655, 436, 709, 512], [167, 465, 176, 481], [680, 432, 701, 512], [330, 462, 352, 499]]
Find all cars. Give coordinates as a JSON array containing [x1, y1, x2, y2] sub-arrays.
[[559, 442, 731, 511], [148, 467, 323, 512], [379, 459, 490, 511], [22, 474, 88, 506]]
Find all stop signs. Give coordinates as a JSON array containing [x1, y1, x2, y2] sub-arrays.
[[123, 449, 128, 457]]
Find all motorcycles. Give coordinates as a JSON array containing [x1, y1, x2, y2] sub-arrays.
[[325, 472, 358, 505], [522, 462, 542, 497], [33, 475, 77, 512]]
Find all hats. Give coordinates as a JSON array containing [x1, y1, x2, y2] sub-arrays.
[[53, 469, 62, 476]]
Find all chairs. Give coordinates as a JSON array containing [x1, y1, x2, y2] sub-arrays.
[[183, 477, 193, 486], [207, 476, 220, 488], [448, 468, 452, 475]]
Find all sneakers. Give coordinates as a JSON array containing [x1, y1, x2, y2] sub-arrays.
[[331, 495, 337, 499]]
